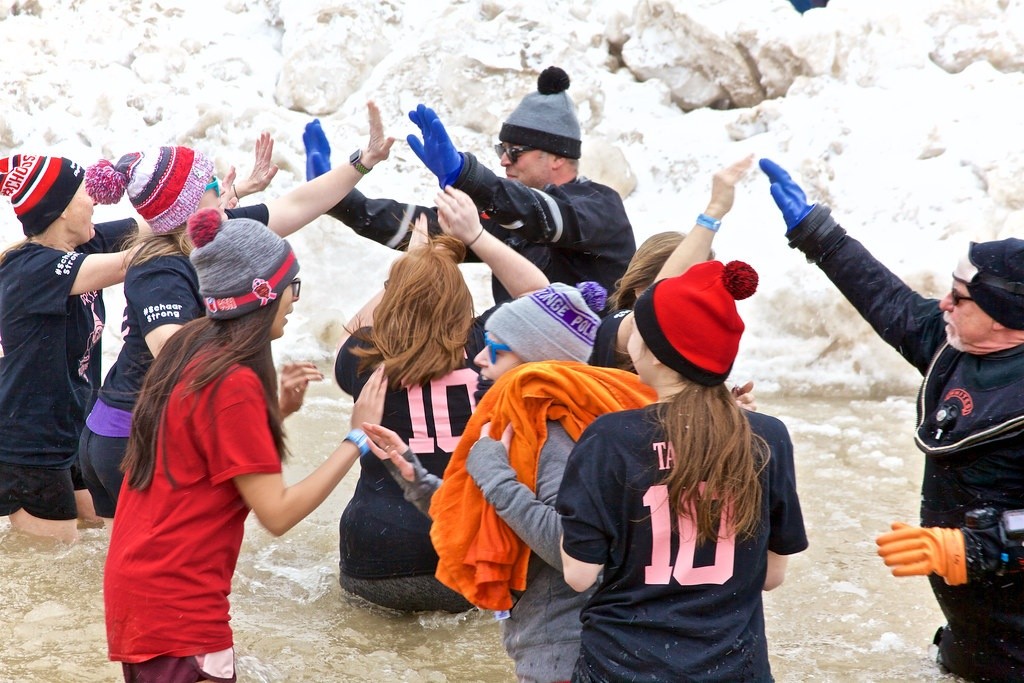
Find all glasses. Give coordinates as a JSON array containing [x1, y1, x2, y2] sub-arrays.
[[384, 280, 390, 289], [483, 332, 512, 364], [951, 240, 982, 283], [291, 277, 301, 296], [494, 142, 534, 164], [949, 287, 972, 306], [205, 174, 220, 196]]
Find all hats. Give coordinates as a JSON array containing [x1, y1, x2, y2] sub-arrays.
[[633, 260, 759, 386], [85, 145, 214, 234], [188, 208, 300, 318], [968, 236, 1024, 331], [498, 65, 581, 159], [485, 281, 608, 364], [0, 153, 85, 236]]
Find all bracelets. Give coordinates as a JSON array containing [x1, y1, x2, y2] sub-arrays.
[[233, 184, 240, 199], [343, 428, 373, 457], [696, 213, 721, 232], [469, 228, 484, 247]]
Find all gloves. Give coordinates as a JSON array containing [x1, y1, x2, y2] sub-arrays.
[[874, 520, 968, 584], [302, 118, 332, 181], [407, 104, 461, 188], [759, 156, 816, 231]]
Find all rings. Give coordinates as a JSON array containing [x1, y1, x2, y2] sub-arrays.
[[384, 446, 389, 450]]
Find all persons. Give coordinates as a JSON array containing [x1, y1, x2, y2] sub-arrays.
[[554, 260, 809, 683], [359, 281, 604, 683], [303, 66, 637, 319], [586, 153, 757, 412], [0, 132, 279, 544], [103, 207, 388, 683], [759, 157, 1024, 683], [334, 184, 551, 614], [78, 100, 397, 519]]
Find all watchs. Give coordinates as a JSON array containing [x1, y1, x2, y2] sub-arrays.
[[350, 148, 373, 174]]
